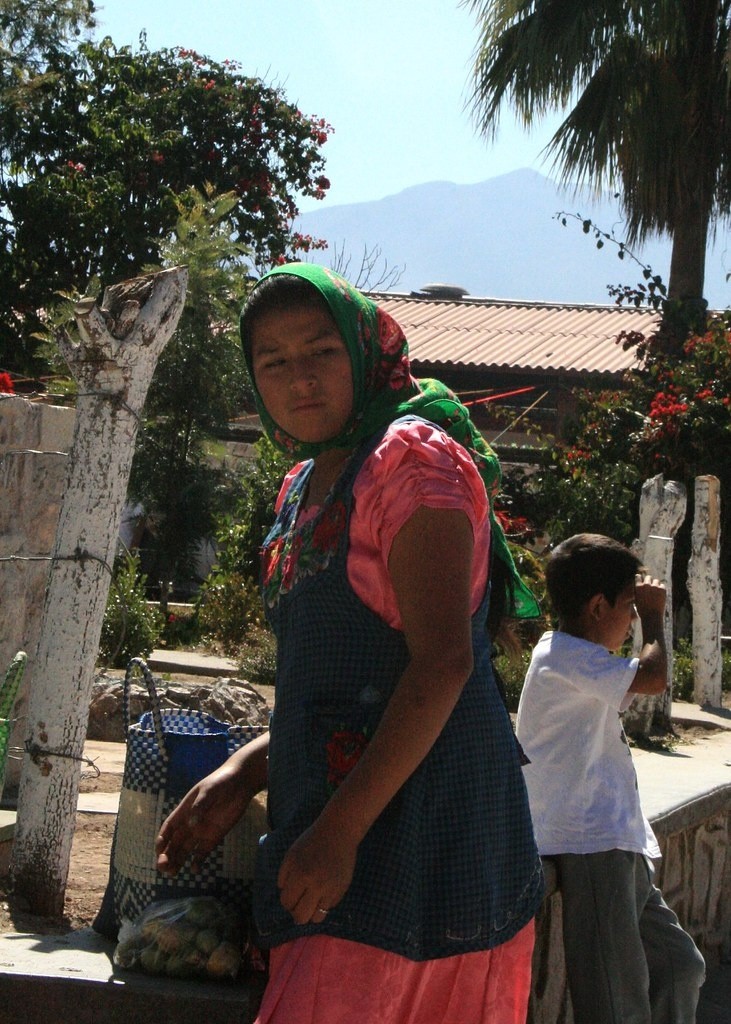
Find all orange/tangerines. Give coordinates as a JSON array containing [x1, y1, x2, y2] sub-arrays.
[[115, 898, 258, 981]]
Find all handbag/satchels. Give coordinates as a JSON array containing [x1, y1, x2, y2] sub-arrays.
[[92, 658, 271, 938], [113, 897, 245, 977]]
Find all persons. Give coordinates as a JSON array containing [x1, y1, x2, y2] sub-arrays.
[[515, 532, 705, 1024], [155, 263, 547, 1024]]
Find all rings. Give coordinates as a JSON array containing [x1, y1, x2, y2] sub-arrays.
[[318, 908, 328, 914]]
